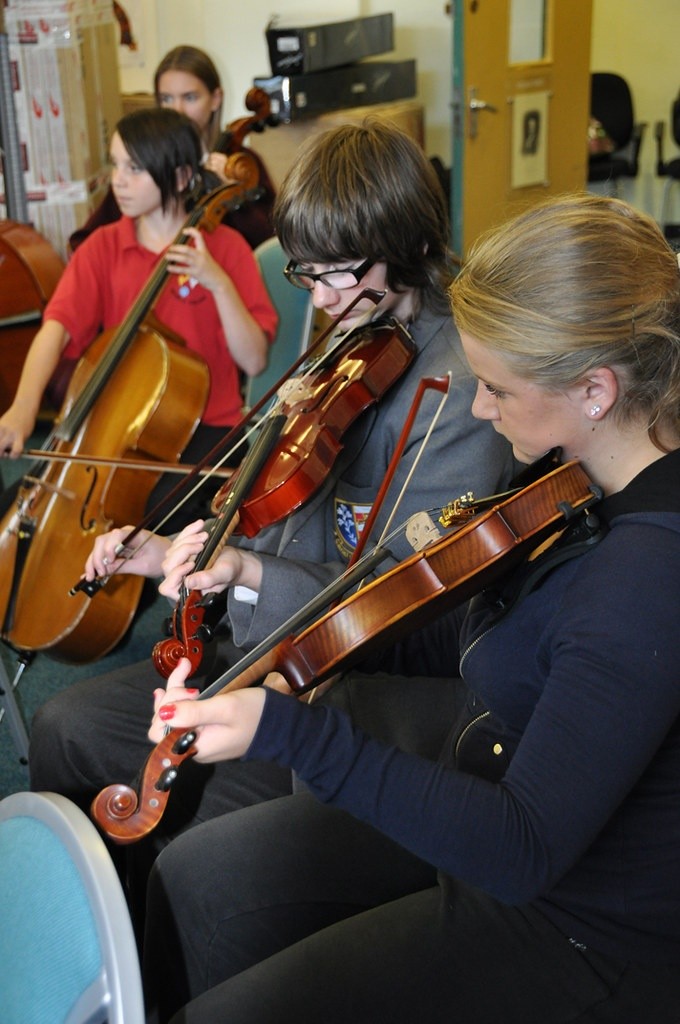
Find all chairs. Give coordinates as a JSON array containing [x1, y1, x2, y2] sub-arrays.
[[586, 71, 680, 253]]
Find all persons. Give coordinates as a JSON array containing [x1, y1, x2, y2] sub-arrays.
[[0, 107, 277, 535], [29, 109, 547, 907], [148, 192, 680, 1024], [71, 46, 279, 251]]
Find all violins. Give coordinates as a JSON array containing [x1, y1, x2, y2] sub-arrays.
[[152, 314, 421, 679], [90, 457, 596, 842]]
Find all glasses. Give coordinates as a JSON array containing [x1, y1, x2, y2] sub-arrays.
[[282, 251, 383, 289]]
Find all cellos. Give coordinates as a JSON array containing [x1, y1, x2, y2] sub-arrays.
[[212, 85, 285, 165], [1, 151, 264, 693], [0, 0, 79, 417]]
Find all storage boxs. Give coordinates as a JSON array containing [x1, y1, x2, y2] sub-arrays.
[[243, 12, 425, 200]]
